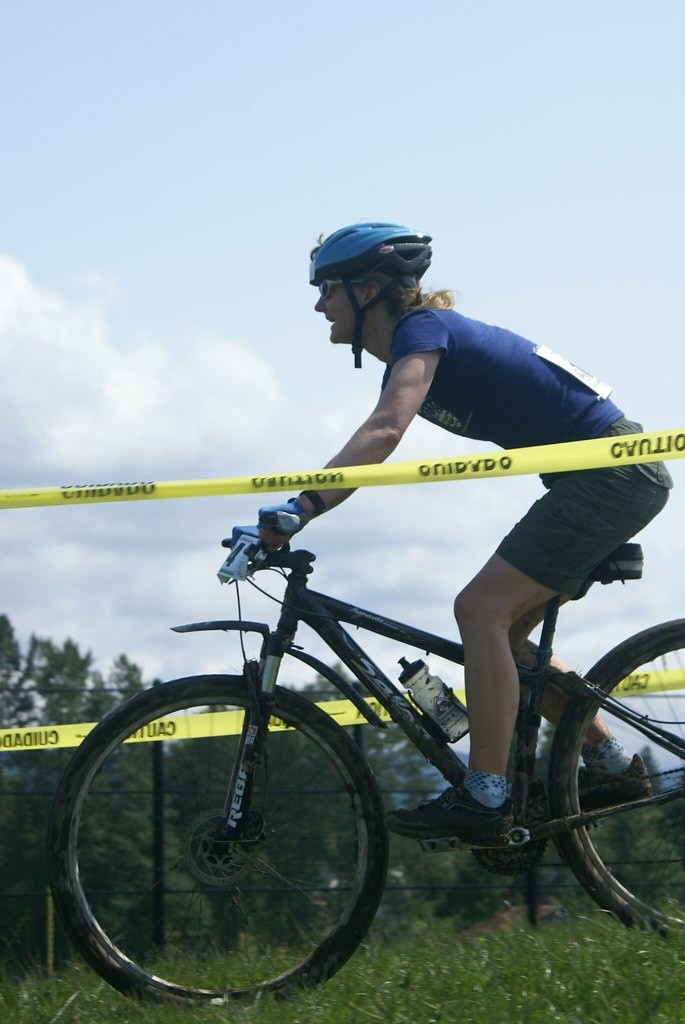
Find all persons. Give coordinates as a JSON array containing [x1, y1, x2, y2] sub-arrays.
[[256, 222, 673, 847]]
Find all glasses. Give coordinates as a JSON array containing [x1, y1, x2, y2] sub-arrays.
[[319, 279, 370, 300]]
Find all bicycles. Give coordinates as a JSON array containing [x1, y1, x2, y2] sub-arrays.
[[46, 505, 685, 1010]]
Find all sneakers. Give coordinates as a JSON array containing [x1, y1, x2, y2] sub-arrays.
[[575, 753, 652, 811], [383, 785, 512, 843]]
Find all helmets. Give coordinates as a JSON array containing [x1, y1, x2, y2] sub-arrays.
[[309, 222, 433, 286]]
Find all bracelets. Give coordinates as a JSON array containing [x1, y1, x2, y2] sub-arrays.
[[300, 490, 328, 513]]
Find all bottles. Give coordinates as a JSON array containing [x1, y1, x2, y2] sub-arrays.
[[397, 656, 470, 743]]
